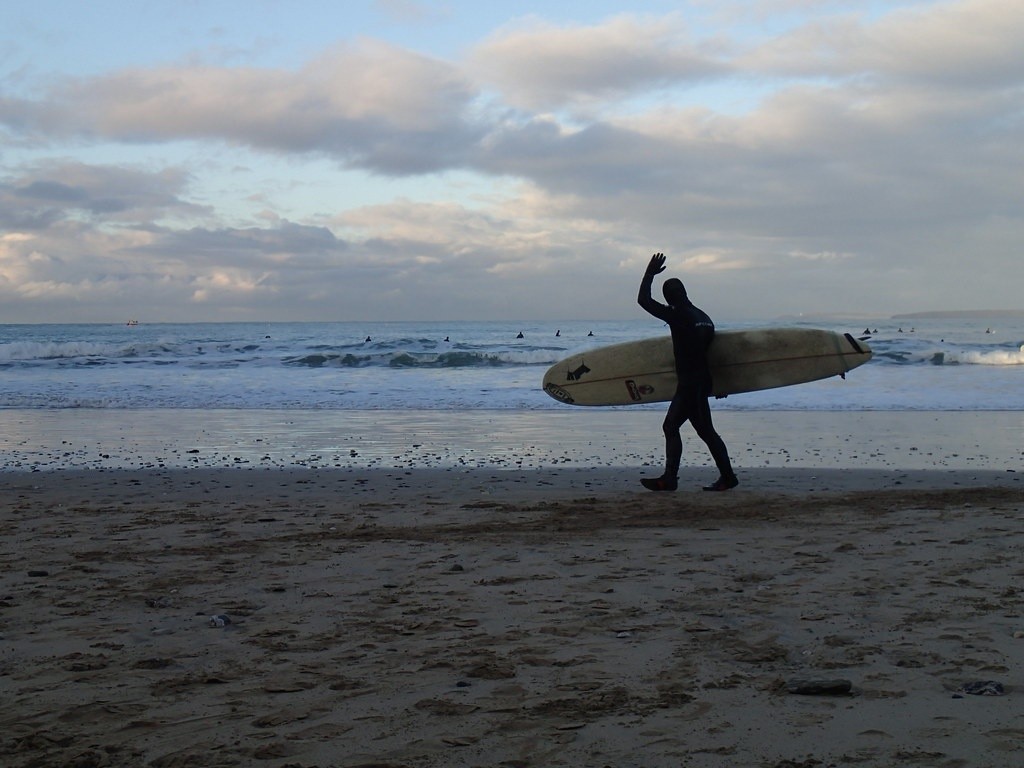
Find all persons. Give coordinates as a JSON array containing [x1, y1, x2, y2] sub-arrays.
[[556, 330, 560, 336], [863, 328, 871, 334], [638, 251, 738, 492], [517, 332, 523, 338], [366, 336, 371, 341], [588, 331, 593, 336], [444, 337, 449, 342]]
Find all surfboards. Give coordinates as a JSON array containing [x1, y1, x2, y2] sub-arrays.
[[543, 325, 873, 406]]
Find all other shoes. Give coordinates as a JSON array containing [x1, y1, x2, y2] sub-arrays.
[[639, 475, 679, 492], [703, 473, 738, 491]]
